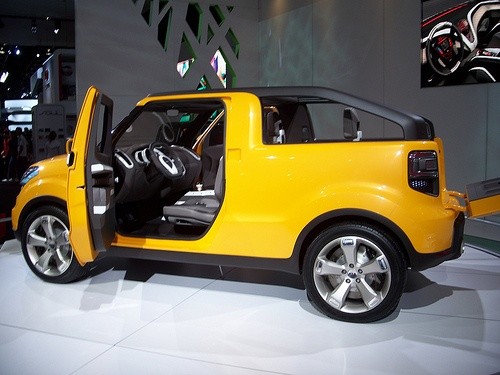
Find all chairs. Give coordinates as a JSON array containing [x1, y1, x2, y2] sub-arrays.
[[343, 107, 362, 142], [163, 106, 286, 228]]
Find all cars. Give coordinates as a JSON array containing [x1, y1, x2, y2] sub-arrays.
[[11, 86, 465, 324]]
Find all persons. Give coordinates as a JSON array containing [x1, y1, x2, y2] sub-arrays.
[[2, 127, 63, 179]]
